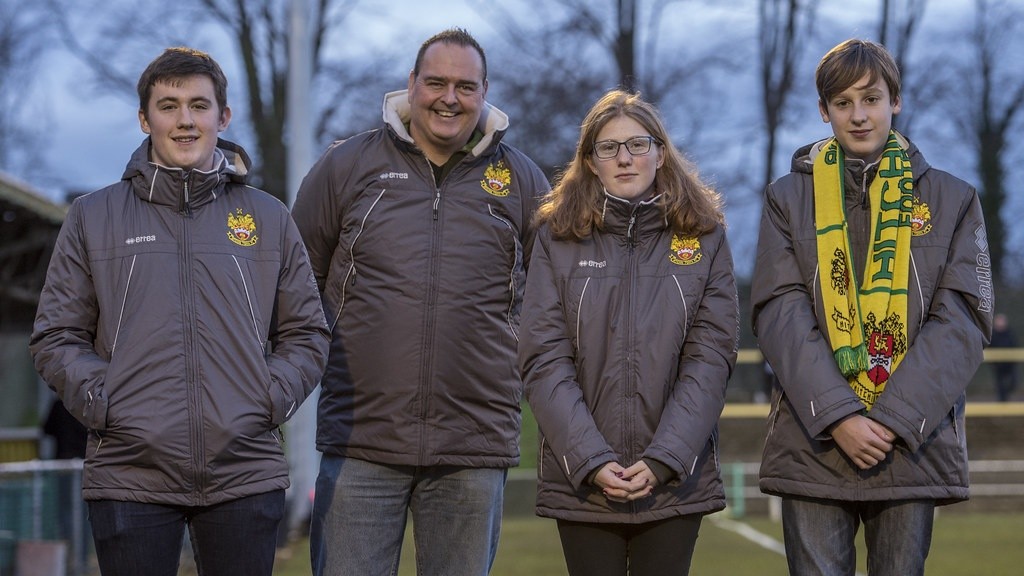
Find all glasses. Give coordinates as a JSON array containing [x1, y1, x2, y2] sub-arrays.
[[588, 136, 660, 159]]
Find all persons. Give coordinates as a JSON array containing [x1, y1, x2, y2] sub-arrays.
[[751, 40, 995, 576], [28, 48, 334, 576], [517, 90, 741, 576], [290, 25, 552, 576], [39, 397, 93, 555]]
